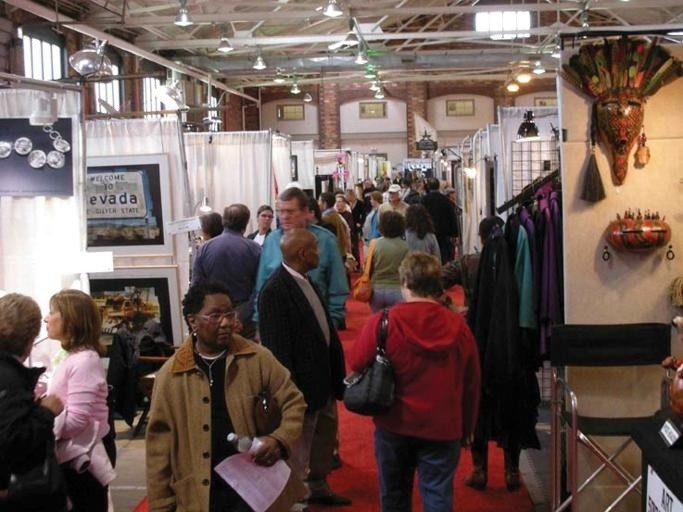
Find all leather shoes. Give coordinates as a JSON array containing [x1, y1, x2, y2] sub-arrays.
[[463, 469, 489, 489], [308, 489, 350, 507], [503, 468, 521, 489]]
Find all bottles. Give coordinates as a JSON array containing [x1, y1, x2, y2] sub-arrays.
[[227, 433, 263, 455]]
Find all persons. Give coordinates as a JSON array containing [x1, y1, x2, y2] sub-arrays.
[[190, 170, 459, 334], [437, 216, 540, 490], [18, 344, 120, 489], [30, 288, 118, 511], [345, 253, 469, 512], [257, 228, 352, 506], [1, 293, 66, 511], [145, 281, 311, 512]]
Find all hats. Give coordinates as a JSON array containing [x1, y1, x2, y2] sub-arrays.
[[388, 184, 400, 193], [479, 216, 504, 238]]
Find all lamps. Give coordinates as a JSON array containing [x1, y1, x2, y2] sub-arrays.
[[28, 90, 57, 126], [518, 111, 538, 138], [69, 40, 112, 77]]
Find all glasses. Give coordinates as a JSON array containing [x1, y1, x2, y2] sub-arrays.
[[198, 312, 236, 324]]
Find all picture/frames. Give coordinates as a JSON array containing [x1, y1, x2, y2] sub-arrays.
[[86, 153, 174, 258], [88, 265, 184, 357], [359, 101, 386, 119], [277, 104, 305, 121], [447, 99, 474, 116]]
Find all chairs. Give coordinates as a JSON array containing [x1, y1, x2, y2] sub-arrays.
[[547, 323, 672, 512]]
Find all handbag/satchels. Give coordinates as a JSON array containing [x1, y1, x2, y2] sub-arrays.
[[252, 389, 283, 435], [352, 275, 372, 302], [5, 467, 69, 512], [342, 355, 398, 415]]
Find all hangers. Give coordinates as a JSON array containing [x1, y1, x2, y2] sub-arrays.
[[505, 173, 553, 216]]
